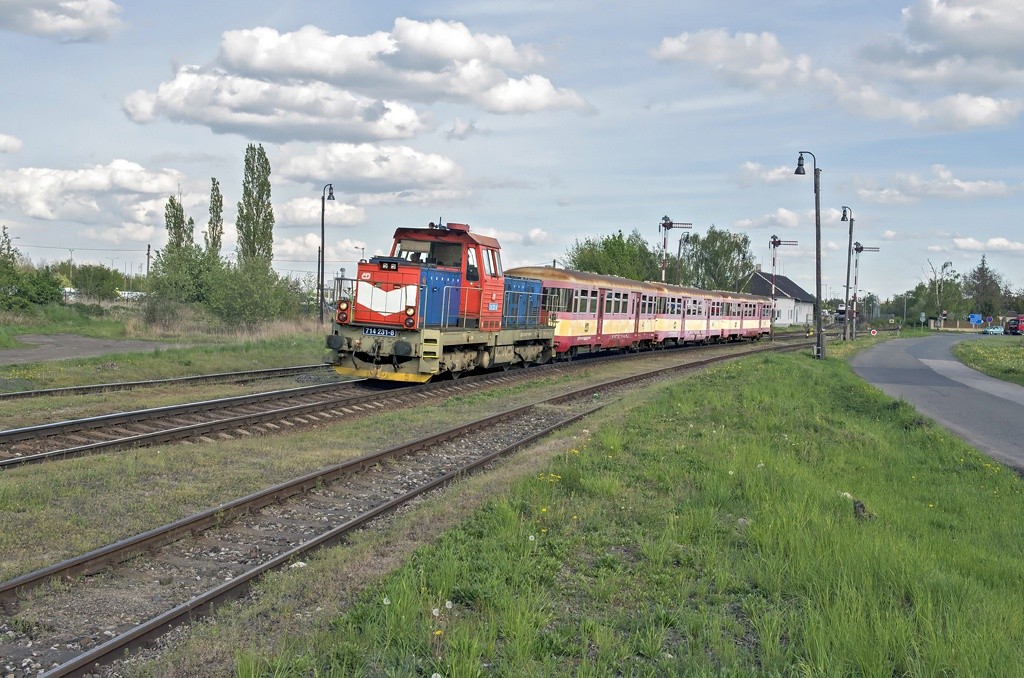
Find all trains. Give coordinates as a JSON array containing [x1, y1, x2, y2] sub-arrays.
[[321, 217, 775, 384]]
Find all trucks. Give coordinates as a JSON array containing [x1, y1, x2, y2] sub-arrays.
[[1006, 314, 1024, 335]]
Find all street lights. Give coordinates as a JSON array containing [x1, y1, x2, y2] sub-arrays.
[[354, 246, 364, 258], [900, 294, 906, 321], [319, 183, 335, 323], [68, 249, 74, 284], [841, 205, 855, 340], [858, 289, 867, 321], [794, 150, 826, 360], [677, 232, 689, 284]]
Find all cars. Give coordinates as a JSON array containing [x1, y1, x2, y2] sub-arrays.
[[982, 326, 1004, 335]]
